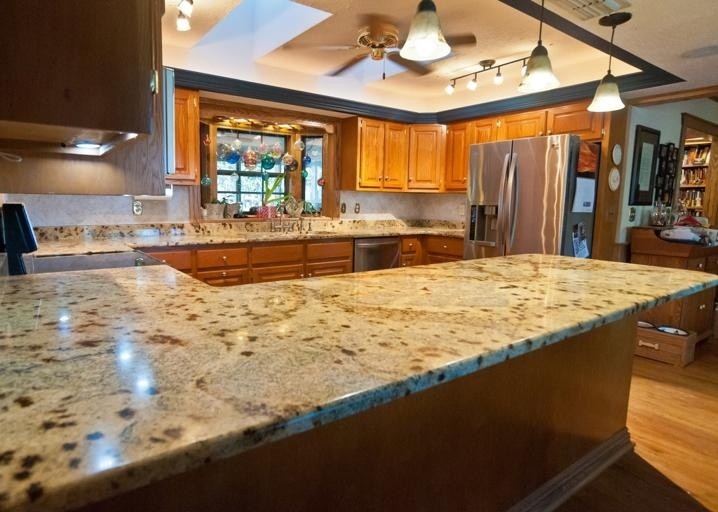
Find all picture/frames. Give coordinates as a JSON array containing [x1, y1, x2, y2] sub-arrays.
[[627, 124, 660, 209]]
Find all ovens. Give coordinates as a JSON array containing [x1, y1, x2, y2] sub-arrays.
[[351, 237, 403, 273]]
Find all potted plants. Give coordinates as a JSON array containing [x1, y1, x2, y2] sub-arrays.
[[257, 167, 288, 219]]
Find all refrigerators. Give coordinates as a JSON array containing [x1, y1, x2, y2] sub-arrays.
[[467, 133, 600, 260]]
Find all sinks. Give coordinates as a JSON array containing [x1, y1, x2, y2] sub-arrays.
[[235, 232, 292, 238], [292, 229, 337, 235]]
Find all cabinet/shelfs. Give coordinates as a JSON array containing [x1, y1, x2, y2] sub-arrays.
[[337, 95, 627, 198], [0, 0, 157, 158], [623, 221, 718, 342], [192, 242, 250, 288], [421, 232, 466, 267], [247, 238, 354, 284], [165, 87, 202, 188], [397, 235, 421, 266], [677, 140, 712, 212], [0, 0, 167, 197], [140, 247, 195, 279]]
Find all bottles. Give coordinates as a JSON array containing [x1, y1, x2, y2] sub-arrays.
[[683, 189, 702, 207], [679, 169, 687, 185], [688, 170, 695, 184], [691, 155, 705, 165], [648, 201, 669, 226], [682, 149, 688, 166]]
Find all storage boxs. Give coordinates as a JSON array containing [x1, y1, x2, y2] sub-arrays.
[[633, 325, 697, 368]]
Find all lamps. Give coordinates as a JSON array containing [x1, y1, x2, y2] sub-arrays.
[[173, 0, 194, 36], [517, 1, 561, 91], [583, 9, 632, 113], [441, 54, 530, 98], [396, 0, 452, 64]]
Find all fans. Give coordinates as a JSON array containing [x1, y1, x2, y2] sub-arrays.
[[279, 12, 476, 79]]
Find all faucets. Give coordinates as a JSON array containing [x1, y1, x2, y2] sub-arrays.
[[298, 217, 304, 231], [278, 199, 286, 232]]
